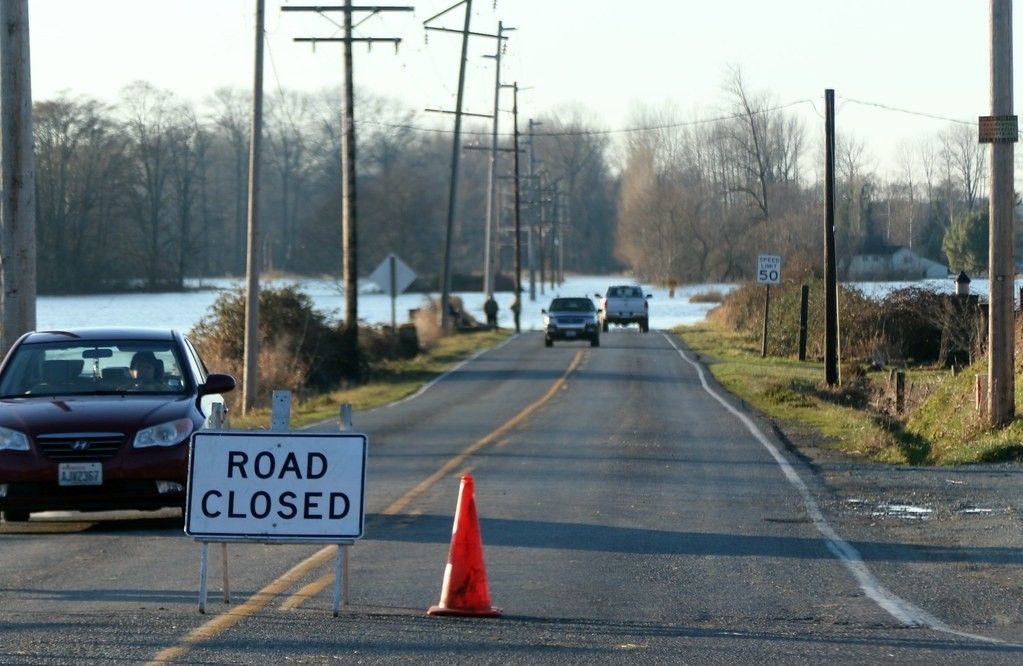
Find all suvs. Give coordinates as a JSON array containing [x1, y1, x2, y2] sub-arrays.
[[541, 296, 603, 346]]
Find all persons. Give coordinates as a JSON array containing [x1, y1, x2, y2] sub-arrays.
[[129, 351, 159, 389], [484, 295, 498, 324]]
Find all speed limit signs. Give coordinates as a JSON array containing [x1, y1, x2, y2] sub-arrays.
[[758, 254, 782, 285]]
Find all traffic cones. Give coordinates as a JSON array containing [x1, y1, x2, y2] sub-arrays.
[[425, 472, 505, 615]]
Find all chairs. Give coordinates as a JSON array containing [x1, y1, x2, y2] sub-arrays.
[[97, 368, 131, 392], [30, 358, 83, 394]]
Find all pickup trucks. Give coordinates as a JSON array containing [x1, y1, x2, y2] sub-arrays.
[[594, 284, 653, 333]]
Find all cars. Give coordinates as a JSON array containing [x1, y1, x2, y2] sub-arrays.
[[1, 327, 238, 520]]
